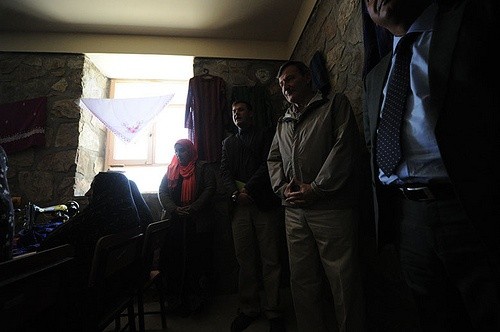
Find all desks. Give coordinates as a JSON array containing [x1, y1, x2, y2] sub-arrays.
[[0, 224, 80, 300]]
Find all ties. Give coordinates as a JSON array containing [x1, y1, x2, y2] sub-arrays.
[[377, 32, 418, 178]]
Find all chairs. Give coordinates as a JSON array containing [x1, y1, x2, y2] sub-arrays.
[[87, 219, 171, 332]]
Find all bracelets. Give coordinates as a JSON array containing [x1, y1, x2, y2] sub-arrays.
[[310, 181, 320, 196]]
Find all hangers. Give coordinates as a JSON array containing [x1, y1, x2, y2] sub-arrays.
[[190, 69, 223, 80]]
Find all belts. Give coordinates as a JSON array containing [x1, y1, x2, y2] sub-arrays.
[[399, 182, 451, 201]]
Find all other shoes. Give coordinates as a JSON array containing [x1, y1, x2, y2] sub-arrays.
[[269, 317, 285, 332], [231, 312, 254, 332]]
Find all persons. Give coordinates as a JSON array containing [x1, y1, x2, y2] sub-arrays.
[[41, 172, 155, 332], [0, 144, 15, 260], [158, 140, 217, 319], [266, 61, 365, 332], [217, 98, 287, 332], [365, 0, 500, 332]]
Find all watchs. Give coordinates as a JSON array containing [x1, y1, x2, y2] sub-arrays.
[[231, 190, 238, 206]]
